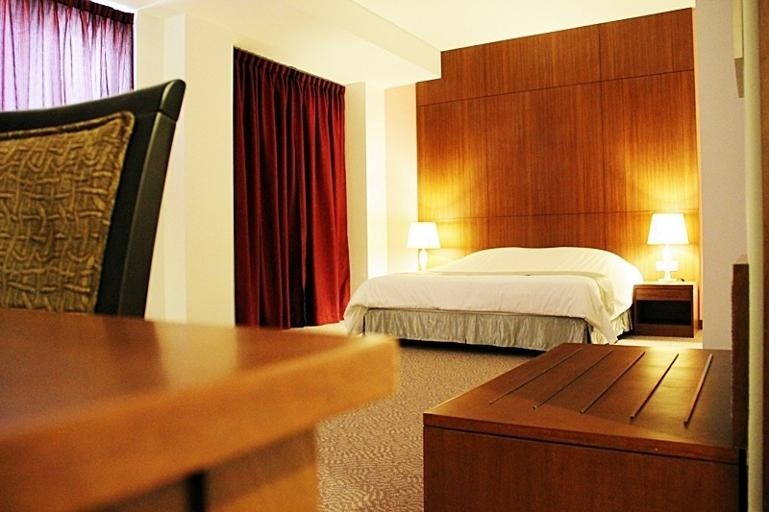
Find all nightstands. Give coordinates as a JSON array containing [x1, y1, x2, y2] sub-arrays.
[[632, 278, 700, 340]]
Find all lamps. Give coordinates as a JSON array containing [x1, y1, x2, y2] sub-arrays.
[[647, 211, 690, 283]]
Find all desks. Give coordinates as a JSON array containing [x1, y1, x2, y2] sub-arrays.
[[0, 303, 402, 511], [424, 336, 742, 512]]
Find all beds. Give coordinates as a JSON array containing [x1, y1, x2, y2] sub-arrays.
[[360, 246, 637, 358]]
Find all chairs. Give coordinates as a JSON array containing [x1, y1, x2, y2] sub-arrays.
[[0, 77, 188, 319]]
